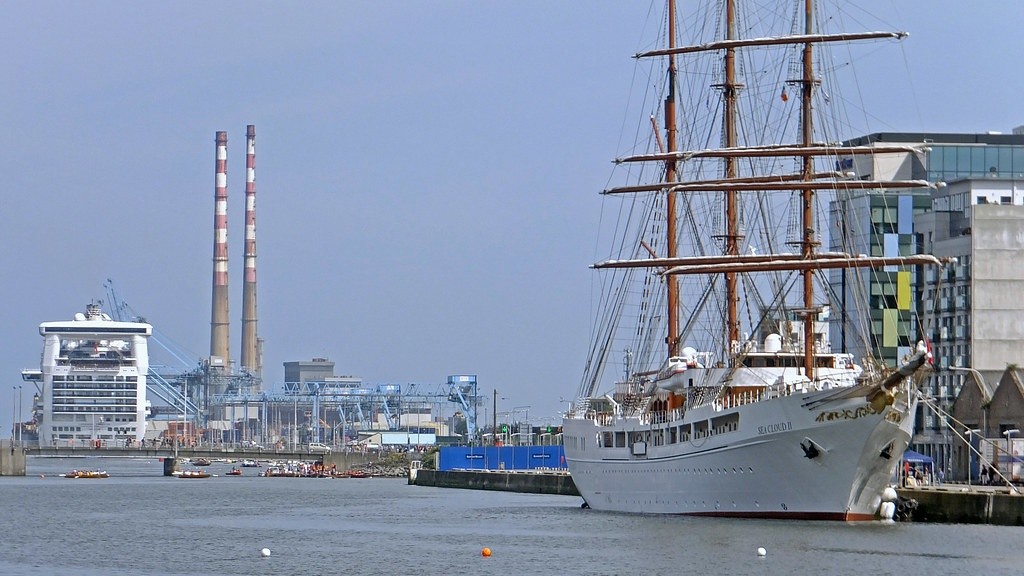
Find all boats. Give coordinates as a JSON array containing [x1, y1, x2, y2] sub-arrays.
[[225, 466, 242, 475], [65, 472, 110, 478], [171, 469, 211, 478], [240, 460, 261, 467], [192, 458, 212, 466]]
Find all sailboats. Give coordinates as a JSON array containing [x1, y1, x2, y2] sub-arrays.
[[559, 1, 960, 521]]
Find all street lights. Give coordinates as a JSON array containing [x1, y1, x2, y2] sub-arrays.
[[19, 385, 23, 441], [13, 386, 16, 440]]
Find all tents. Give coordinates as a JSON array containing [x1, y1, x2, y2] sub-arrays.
[[897, 450, 934, 488]]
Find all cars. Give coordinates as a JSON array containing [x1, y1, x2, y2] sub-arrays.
[[308, 442, 331, 453]]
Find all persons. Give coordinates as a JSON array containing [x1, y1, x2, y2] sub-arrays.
[[494, 437, 503, 446], [980, 464, 989, 485], [141, 437, 147, 448], [125, 437, 132, 448], [151, 437, 156, 448]]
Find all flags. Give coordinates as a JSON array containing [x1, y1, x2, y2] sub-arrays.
[[926, 334, 934, 366]]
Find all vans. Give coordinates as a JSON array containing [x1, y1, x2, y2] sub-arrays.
[[241, 440, 264, 452]]
